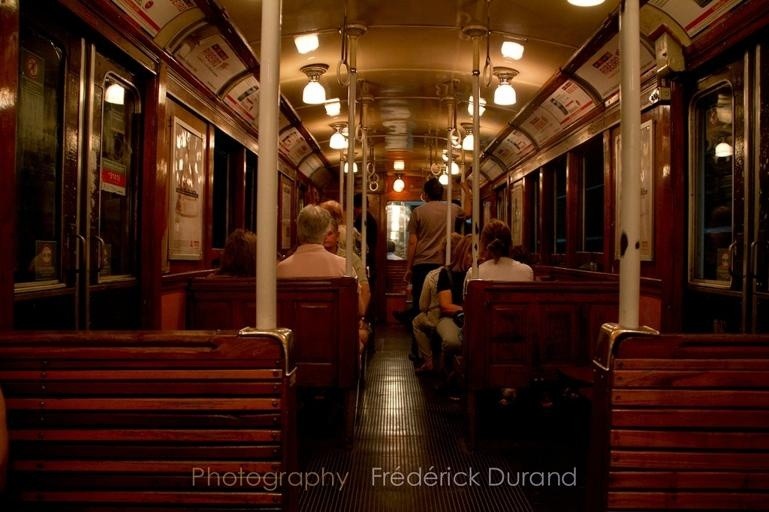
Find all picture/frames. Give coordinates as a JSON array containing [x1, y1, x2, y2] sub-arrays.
[[613, 119, 657, 261], [169, 116, 205, 262], [510, 184, 524, 248]]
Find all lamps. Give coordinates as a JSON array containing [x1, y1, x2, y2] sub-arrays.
[[292, 31, 528, 193]]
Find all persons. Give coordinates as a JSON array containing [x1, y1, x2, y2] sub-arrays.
[[433, 231, 474, 401], [461, 219, 537, 412], [277, 203, 372, 352], [404, 178, 474, 362], [316, 193, 377, 320], [213, 228, 257, 278], [412, 232, 464, 374]]
